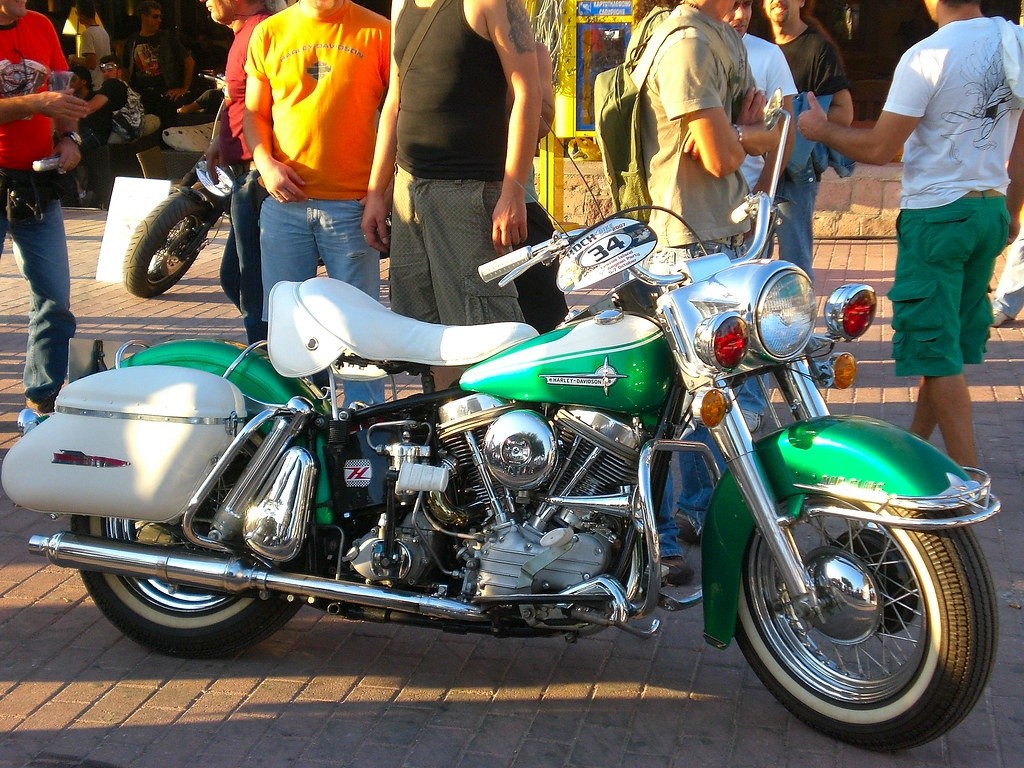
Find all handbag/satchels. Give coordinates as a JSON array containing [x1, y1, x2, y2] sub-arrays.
[[7, 170, 67, 226]]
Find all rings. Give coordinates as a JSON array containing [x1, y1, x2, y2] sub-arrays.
[[274, 195, 281, 199]]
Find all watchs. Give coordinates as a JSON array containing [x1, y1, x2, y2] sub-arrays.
[[60, 131, 83, 151]]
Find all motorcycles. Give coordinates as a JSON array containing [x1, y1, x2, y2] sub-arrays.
[[0, 89, 1002, 753], [122, 68, 392, 299]]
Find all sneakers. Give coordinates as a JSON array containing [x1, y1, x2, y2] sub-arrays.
[[17, 408, 42, 437]]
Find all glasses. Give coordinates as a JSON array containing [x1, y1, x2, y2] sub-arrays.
[[150, 13, 161, 19], [71, 77, 80, 82], [100, 64, 116, 69]]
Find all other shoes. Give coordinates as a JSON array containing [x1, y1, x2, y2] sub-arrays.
[[674, 510, 701, 544], [992, 308, 1011, 328], [662, 556, 695, 584]]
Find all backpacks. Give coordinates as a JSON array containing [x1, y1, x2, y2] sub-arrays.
[[594, 15, 742, 225], [112, 79, 145, 142]]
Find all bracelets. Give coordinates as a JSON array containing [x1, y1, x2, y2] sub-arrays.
[[732, 123, 742, 143]]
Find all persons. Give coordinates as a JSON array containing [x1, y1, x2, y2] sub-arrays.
[[798, 0, 1024, 485], [200, 0, 857, 580], [0, 0, 90, 417], [38, 0, 222, 207], [984, 232, 1024, 329]]
